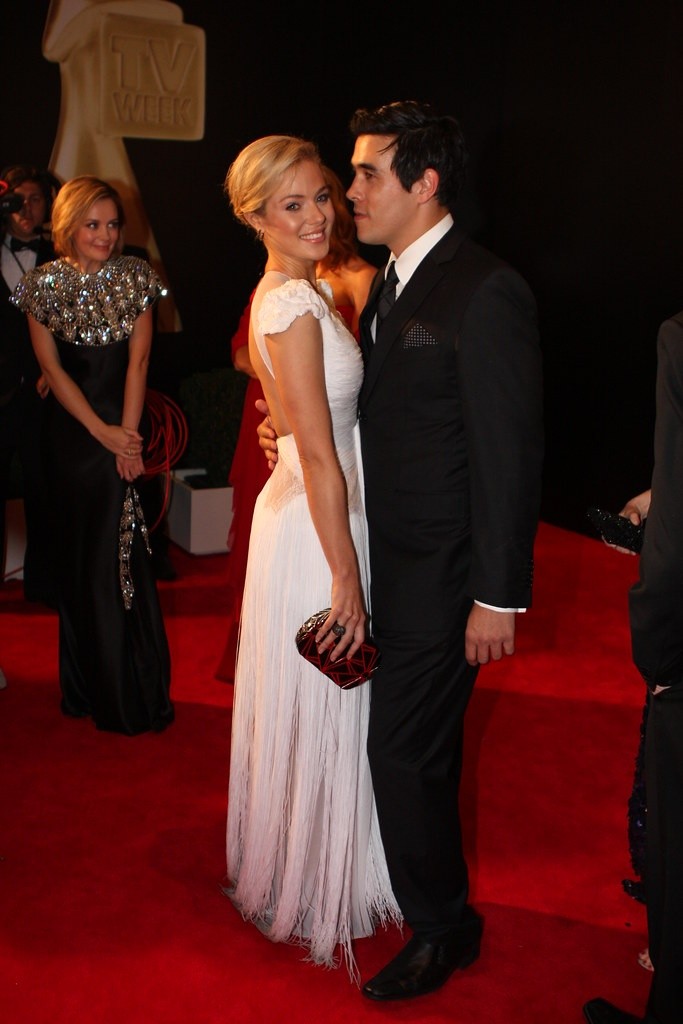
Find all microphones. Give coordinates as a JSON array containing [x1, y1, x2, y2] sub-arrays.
[[33, 225, 52, 235]]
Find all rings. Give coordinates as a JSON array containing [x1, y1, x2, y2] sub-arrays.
[[129, 449, 131, 456], [331, 624, 346, 636]]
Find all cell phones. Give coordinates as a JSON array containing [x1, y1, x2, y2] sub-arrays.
[[587, 504, 644, 552]]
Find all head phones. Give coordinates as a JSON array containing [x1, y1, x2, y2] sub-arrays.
[[0, 165, 55, 223]]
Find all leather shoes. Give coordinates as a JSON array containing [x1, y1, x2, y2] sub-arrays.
[[583, 997, 643, 1024], [362, 903, 482, 1001]]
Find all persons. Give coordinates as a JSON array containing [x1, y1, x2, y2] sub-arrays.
[[582, 316, 683, 1023], [254, 103, 531, 1000], [214, 172, 376, 683], [0, 168, 74, 608], [12, 177, 175, 734], [226, 138, 408, 979]]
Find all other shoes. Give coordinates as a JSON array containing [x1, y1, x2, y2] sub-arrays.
[[157, 554, 178, 580], [23, 589, 58, 611]]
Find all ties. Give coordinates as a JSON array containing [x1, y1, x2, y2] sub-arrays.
[[374, 260, 400, 340]]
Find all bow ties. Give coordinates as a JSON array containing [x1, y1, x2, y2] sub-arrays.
[[9, 236, 40, 253]]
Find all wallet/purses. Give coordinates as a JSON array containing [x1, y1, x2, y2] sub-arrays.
[[295, 607, 380, 690]]
